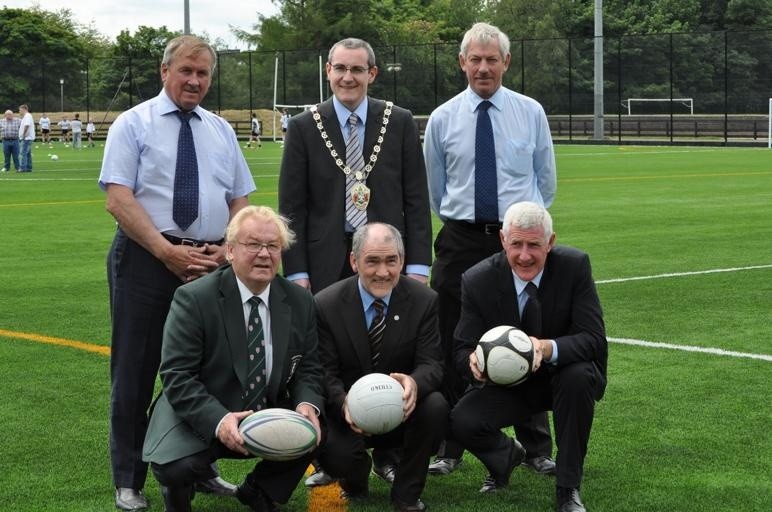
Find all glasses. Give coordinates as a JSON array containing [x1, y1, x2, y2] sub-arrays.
[[326, 61, 381, 74], [230, 240, 285, 256]]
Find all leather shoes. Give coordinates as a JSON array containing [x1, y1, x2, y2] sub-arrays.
[[370, 459, 397, 485], [304, 470, 336, 488], [426, 455, 464, 475], [556, 485, 588, 512], [114, 486, 149, 511], [479, 436, 526, 495], [195, 474, 239, 497], [234, 481, 276, 512], [529, 456, 556, 474], [390, 488, 426, 512], [338, 489, 369, 504]]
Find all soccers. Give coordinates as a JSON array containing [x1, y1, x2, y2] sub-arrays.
[[347, 373, 407, 435], [51, 154, 57, 161], [34, 141, 104, 151], [475, 325, 534, 386]]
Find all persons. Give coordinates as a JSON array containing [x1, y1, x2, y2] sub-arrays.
[[280, 109, 292, 148], [100, 35, 257, 510], [448, 201, 607, 512], [244, 113, 262, 149], [0, 105, 96, 173], [140, 205, 329, 511], [278, 37, 432, 487], [423, 20, 557, 476], [313, 223, 450, 512]]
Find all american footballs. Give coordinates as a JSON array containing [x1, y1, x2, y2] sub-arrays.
[[238, 409, 318, 461]]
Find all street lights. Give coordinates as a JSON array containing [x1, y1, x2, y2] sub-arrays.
[[388, 63, 402, 103], [59, 77, 65, 112]]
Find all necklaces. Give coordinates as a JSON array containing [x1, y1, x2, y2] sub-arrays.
[[310, 101, 394, 211]]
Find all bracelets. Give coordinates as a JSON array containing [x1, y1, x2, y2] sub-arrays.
[[540, 342, 546, 360]]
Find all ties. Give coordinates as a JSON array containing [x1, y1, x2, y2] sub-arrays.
[[366, 299, 387, 376], [474, 100, 501, 227], [172, 109, 200, 233], [345, 112, 368, 232], [520, 282, 542, 340], [241, 294, 267, 412]]
